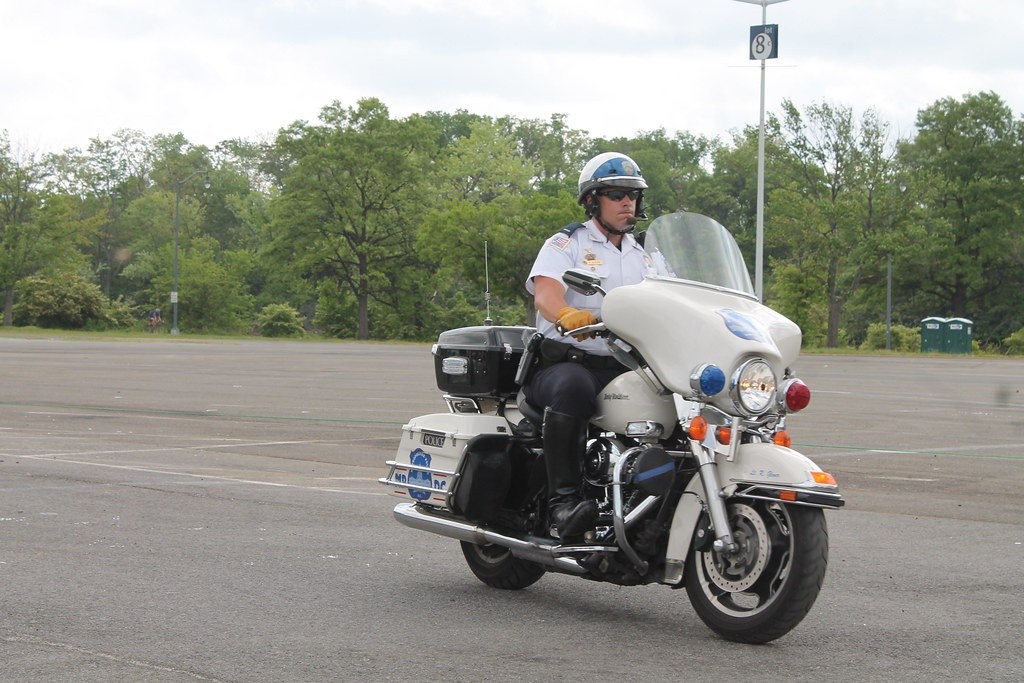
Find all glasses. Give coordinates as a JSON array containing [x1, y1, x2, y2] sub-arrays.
[[596, 189, 639, 201]]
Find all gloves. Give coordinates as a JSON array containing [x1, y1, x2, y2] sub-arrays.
[[554, 306, 597, 342]]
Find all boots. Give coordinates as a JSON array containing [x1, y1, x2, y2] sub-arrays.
[[542, 408, 599, 546]]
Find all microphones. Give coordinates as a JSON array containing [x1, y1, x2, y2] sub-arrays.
[[627, 215, 648, 224]]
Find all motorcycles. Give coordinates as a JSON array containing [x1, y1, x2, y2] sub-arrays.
[[374, 211, 849, 645]]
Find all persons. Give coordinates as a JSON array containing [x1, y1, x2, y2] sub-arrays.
[[522, 151, 678, 542]]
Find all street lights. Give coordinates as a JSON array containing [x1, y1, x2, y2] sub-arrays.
[[735, 0, 792, 301], [169, 171, 211, 333], [884, 176, 908, 351]]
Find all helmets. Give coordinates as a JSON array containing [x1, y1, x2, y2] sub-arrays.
[[577, 151, 649, 207]]
